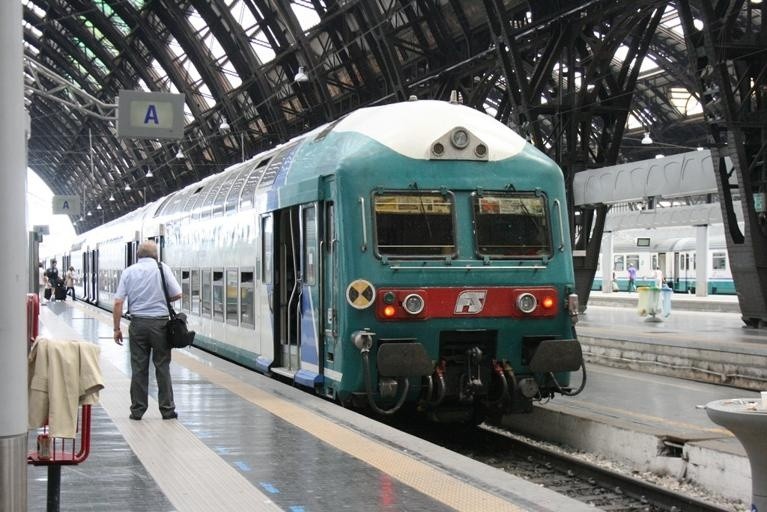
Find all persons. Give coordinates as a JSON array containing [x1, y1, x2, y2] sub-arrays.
[[655, 267, 665, 288], [628, 263, 636, 292], [39, 259, 77, 305], [113, 240, 182, 419]]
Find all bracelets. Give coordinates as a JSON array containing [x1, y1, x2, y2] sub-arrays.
[[114, 328, 120, 332]]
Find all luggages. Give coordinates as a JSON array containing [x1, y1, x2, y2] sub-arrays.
[[54, 287, 67, 301]]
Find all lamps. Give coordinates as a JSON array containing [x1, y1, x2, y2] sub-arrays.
[[176, 149, 184, 158], [641, 132, 653, 145], [293, 65, 309, 82], [218, 117, 231, 129], [70, 167, 154, 227]]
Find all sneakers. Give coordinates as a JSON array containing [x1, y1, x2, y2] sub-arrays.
[[129, 413, 143, 420], [163, 412, 178, 419]]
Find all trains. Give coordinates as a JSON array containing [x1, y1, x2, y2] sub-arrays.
[[45, 95, 588, 441], [571, 222, 744, 298]]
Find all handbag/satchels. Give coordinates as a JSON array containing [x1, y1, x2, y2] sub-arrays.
[[165, 312, 196, 349]]
